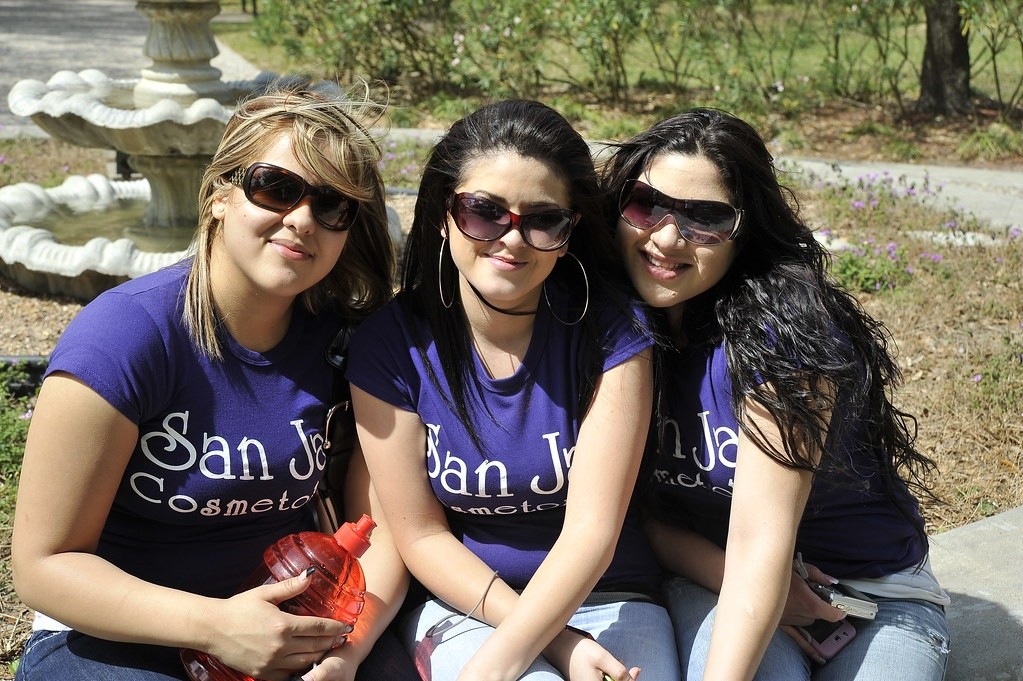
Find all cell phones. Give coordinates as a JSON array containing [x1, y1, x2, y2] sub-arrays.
[[796, 617, 856, 661]]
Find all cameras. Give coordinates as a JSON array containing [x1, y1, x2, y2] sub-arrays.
[[811, 581, 878, 620]]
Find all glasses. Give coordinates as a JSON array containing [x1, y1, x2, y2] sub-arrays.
[[443, 191, 582, 253], [230, 162, 361, 232], [618, 179, 743, 246]]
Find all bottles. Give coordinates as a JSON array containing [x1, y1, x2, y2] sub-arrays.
[[179, 514, 377, 681]]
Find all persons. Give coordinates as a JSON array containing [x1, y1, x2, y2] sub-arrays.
[[344, 98, 679, 681], [12, 89, 410, 681], [596, 106, 952, 681]]
